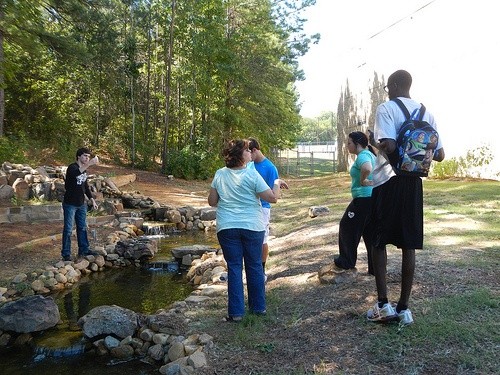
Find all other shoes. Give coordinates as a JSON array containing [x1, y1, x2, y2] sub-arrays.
[[258, 312, 267, 316], [221, 315, 243, 323], [62, 257, 72, 261], [79, 250, 97, 256]]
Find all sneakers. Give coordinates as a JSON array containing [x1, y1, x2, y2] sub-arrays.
[[367, 303, 397, 322], [393, 305, 414, 325]]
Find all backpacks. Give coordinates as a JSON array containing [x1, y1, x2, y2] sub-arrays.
[[387, 97, 439, 177]]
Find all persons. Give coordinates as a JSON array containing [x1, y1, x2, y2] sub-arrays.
[[208, 139, 289, 323], [332, 131, 377, 274], [243, 137, 279, 284], [367, 69, 445, 325], [60, 148, 98, 261]]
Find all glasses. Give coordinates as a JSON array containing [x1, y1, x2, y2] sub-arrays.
[[384, 85, 390, 94]]
